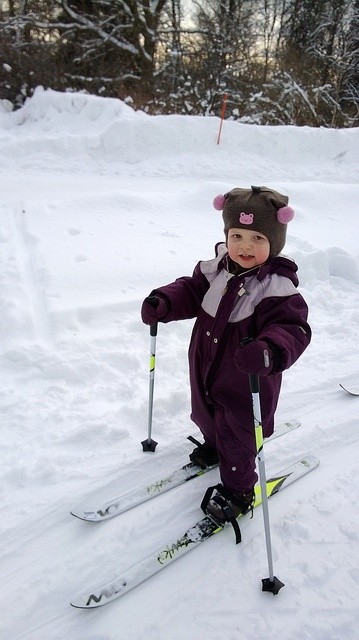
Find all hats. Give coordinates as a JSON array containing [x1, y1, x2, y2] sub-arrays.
[[213, 185, 294, 264]]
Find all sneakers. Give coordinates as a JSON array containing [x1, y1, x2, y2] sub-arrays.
[[187, 436, 219, 467], [207, 488, 254, 523]]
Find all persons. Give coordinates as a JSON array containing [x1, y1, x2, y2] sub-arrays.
[[141, 185, 312, 525]]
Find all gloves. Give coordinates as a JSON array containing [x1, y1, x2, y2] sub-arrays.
[[234, 340, 273, 376], [141, 296, 169, 325]]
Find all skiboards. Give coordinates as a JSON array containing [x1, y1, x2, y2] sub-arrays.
[[68, 418, 320, 609]]
